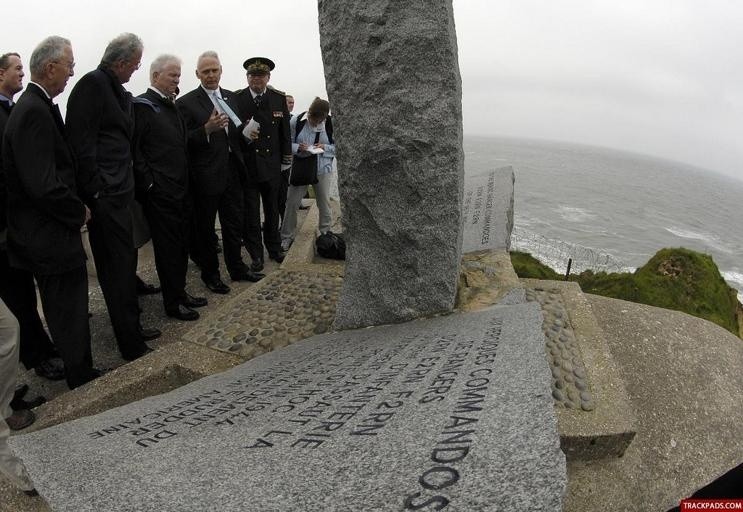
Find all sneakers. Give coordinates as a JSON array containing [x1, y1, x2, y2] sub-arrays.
[[251, 253, 265, 272], [9, 384, 47, 411], [267, 249, 287, 262], [136, 274, 161, 295], [280, 238, 293, 252], [5, 408, 36, 431], [23, 488, 40, 498]]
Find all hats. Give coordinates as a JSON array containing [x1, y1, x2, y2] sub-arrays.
[[243, 57, 275, 76]]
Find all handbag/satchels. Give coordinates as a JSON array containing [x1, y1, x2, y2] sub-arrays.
[[316, 230, 346, 260], [291, 154, 319, 187]]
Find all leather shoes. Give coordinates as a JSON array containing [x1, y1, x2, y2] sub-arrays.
[[34, 358, 67, 381], [141, 327, 162, 341], [165, 303, 201, 322], [69, 367, 116, 390], [183, 290, 208, 308], [231, 269, 268, 281], [201, 271, 232, 295]]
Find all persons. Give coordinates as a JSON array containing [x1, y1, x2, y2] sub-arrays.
[[65, 34, 160, 362], [0, 299, 40, 495], [1, 35, 111, 389], [132, 52, 339, 320]]
